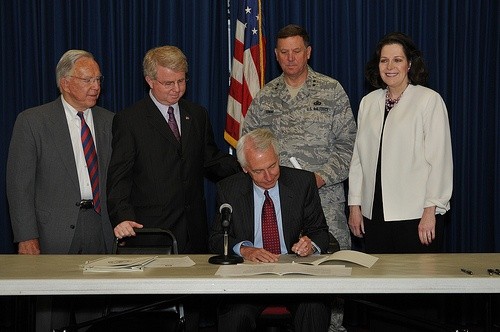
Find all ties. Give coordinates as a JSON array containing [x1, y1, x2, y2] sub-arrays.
[[77, 111, 101, 215], [167, 107, 180, 142], [261, 191, 281, 255]]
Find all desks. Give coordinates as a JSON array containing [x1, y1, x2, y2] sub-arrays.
[[0, 253, 500, 332]]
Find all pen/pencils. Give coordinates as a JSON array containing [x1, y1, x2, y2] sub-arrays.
[[487, 268, 500, 276], [296, 230, 303, 257], [461, 269, 472, 275]]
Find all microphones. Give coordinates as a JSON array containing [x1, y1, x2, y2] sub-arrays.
[[219, 203, 233, 227]]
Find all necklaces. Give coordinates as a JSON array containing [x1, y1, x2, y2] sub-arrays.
[[386, 85, 408, 112]]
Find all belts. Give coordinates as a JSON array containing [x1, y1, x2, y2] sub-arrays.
[[80, 200, 94, 211]]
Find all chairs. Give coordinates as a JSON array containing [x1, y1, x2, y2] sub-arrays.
[[104, 227, 179, 321]]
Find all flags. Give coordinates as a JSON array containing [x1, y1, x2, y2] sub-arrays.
[[224, 0, 266, 157]]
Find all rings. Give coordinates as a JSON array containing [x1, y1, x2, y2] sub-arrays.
[[305, 246, 309, 250]]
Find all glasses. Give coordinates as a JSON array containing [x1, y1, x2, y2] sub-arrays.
[[64, 75, 104, 87], [155, 76, 189, 88]]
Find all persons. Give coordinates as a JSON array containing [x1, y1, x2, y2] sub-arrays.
[[106, 46, 241, 332], [208, 129, 330, 332], [348, 34, 454, 332], [241, 24, 357, 252], [6, 48, 120, 332]]
